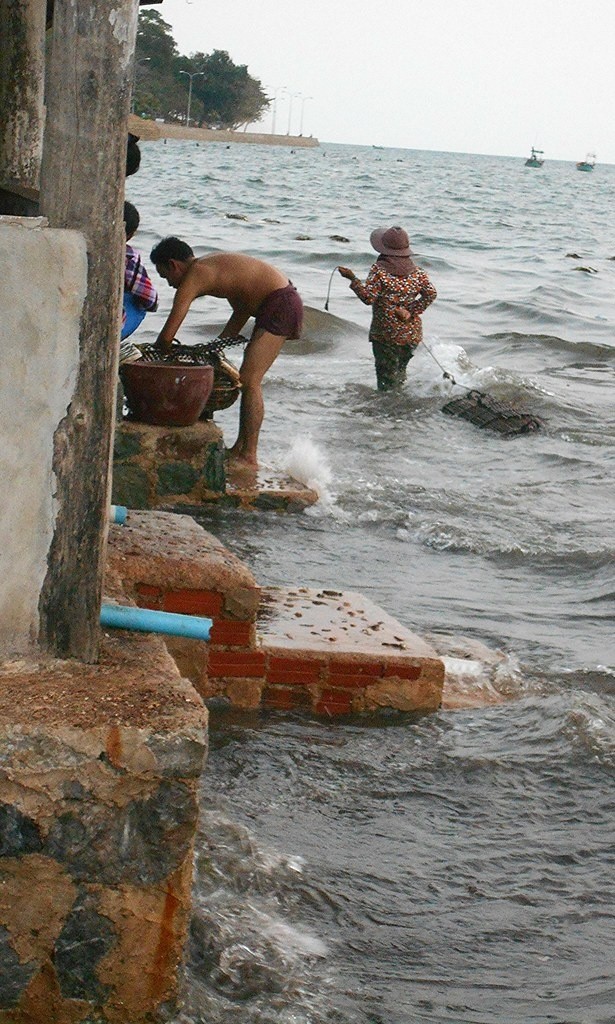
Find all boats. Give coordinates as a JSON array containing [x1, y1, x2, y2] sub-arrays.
[[525, 145, 545, 168], [575, 152, 598, 172]]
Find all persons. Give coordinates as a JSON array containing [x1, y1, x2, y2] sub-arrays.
[[120, 133, 159, 343], [339, 225, 437, 391], [152, 236, 304, 473]]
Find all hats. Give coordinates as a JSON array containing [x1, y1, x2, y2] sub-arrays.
[[370, 226, 414, 257]]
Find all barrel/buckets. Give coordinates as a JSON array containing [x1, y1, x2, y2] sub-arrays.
[[118, 360, 215, 426]]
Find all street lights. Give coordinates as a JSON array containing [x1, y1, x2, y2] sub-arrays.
[[283, 91, 301, 136], [295, 95, 314, 137], [267, 84, 288, 135], [180, 70, 205, 128], [132, 58, 150, 116]]
